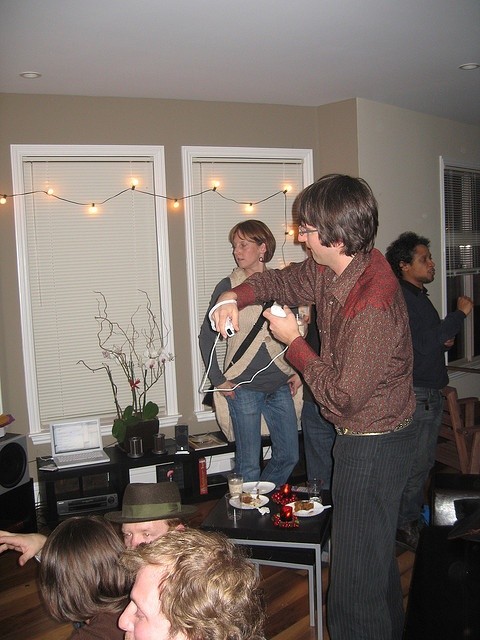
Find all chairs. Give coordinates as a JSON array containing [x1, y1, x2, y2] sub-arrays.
[[436, 386, 480, 474]]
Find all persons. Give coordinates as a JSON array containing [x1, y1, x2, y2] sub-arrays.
[[302, 305, 336, 490], [118, 530, 266, 640], [35, 514, 130, 640], [199, 220, 298, 488], [233, 186, 424, 640], [0, 479, 197, 566], [386, 231, 475, 544]]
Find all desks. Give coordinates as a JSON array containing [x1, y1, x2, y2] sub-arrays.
[[199, 487, 332, 640]]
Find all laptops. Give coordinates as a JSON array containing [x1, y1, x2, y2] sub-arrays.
[[49, 416, 111, 470]]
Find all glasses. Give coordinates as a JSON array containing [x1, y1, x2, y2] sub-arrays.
[[298, 225, 318, 236]]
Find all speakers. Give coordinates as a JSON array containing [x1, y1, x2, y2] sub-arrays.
[[174, 424, 190, 455], [0, 432, 30, 494]]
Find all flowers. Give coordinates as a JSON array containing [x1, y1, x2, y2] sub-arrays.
[[76, 289, 176, 442]]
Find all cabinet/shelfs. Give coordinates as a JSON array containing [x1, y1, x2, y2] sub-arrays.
[[36, 431, 304, 530]]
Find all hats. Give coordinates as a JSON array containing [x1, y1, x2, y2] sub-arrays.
[[104, 481, 199, 522]]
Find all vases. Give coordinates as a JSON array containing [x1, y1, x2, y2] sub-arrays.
[[115, 413, 159, 452]]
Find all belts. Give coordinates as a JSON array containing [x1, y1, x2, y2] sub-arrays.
[[335, 414, 415, 436]]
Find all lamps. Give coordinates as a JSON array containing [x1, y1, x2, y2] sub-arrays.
[[213, 181, 221, 192], [46, 188, 54, 195], [0, 193, 8, 206], [173, 198, 180, 209], [283, 183, 293, 195], [131, 179, 139, 190], [247, 202, 254, 212], [88, 203, 97, 213], [285, 230, 295, 236]]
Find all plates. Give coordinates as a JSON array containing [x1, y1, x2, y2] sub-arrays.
[[239, 482, 275, 494], [229, 494, 269, 510], [285, 500, 323, 518]]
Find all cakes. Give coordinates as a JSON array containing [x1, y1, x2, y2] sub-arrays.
[[239, 493, 258, 506], [294, 501, 314, 513]]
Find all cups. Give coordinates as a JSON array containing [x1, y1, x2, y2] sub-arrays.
[[128, 436, 144, 459], [306, 477, 321, 497], [152, 433, 167, 455], [227, 473, 244, 496], [225, 494, 242, 521]]
